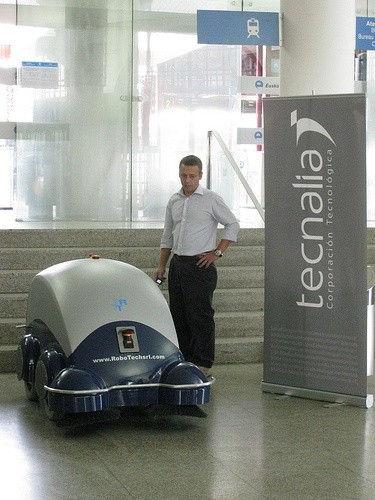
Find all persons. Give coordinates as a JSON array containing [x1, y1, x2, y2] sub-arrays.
[[153, 155, 240, 370]]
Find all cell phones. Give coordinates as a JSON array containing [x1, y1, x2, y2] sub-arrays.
[[155, 276, 164, 287]]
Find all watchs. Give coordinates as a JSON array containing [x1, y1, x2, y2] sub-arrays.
[[214, 248, 223, 258]]
